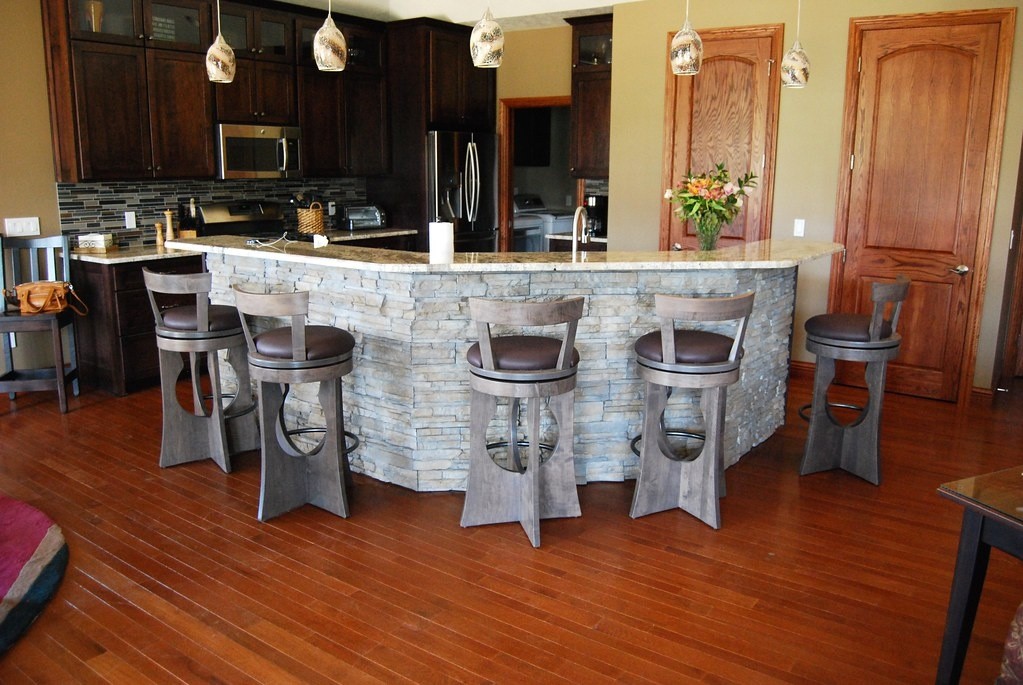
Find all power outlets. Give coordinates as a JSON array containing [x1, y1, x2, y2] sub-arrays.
[[5, 217, 40, 237], [328, 201, 336, 215]]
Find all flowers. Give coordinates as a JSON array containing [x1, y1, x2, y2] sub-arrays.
[[664, 162, 758, 251]]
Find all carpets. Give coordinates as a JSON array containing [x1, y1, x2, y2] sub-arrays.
[[0, 493, 69, 659]]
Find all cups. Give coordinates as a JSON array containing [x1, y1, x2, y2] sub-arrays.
[[83, 0, 104, 32]]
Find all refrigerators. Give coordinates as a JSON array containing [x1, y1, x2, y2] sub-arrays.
[[425, 129, 499, 253]]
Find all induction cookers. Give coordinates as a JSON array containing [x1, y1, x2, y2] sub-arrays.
[[183, 197, 323, 245]]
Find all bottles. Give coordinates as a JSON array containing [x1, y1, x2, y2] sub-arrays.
[[295, 194, 310, 209]]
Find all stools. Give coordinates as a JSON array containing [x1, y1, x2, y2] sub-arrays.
[[459, 297, 585, 548], [799, 274, 911, 485], [234, 283, 359, 521], [142, 266, 262, 473], [630, 292, 755, 530]]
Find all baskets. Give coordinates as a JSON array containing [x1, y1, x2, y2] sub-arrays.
[[297, 202, 324, 235]]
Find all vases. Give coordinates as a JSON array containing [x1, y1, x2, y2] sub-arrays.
[[697, 232, 721, 251]]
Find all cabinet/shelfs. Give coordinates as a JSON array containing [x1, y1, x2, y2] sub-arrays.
[[563, 13, 613, 178], [40, 0, 496, 183], [62, 255, 203, 397]]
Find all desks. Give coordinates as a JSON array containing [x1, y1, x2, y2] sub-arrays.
[[936, 463, 1023, 685]]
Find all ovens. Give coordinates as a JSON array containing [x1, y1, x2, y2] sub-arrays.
[[336, 201, 387, 231]]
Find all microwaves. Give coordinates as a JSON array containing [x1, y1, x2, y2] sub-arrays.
[[214, 122, 305, 182]]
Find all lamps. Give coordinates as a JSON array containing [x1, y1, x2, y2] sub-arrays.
[[313, 0, 347, 72], [470, 7, 504, 68], [206, 0, 236, 83], [670, 0, 703, 76], [781, 0, 810, 88]]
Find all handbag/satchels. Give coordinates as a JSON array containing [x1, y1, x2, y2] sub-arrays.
[[4, 279, 88, 315]]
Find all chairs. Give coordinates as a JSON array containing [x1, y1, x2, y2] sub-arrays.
[[0, 233, 80, 413]]
